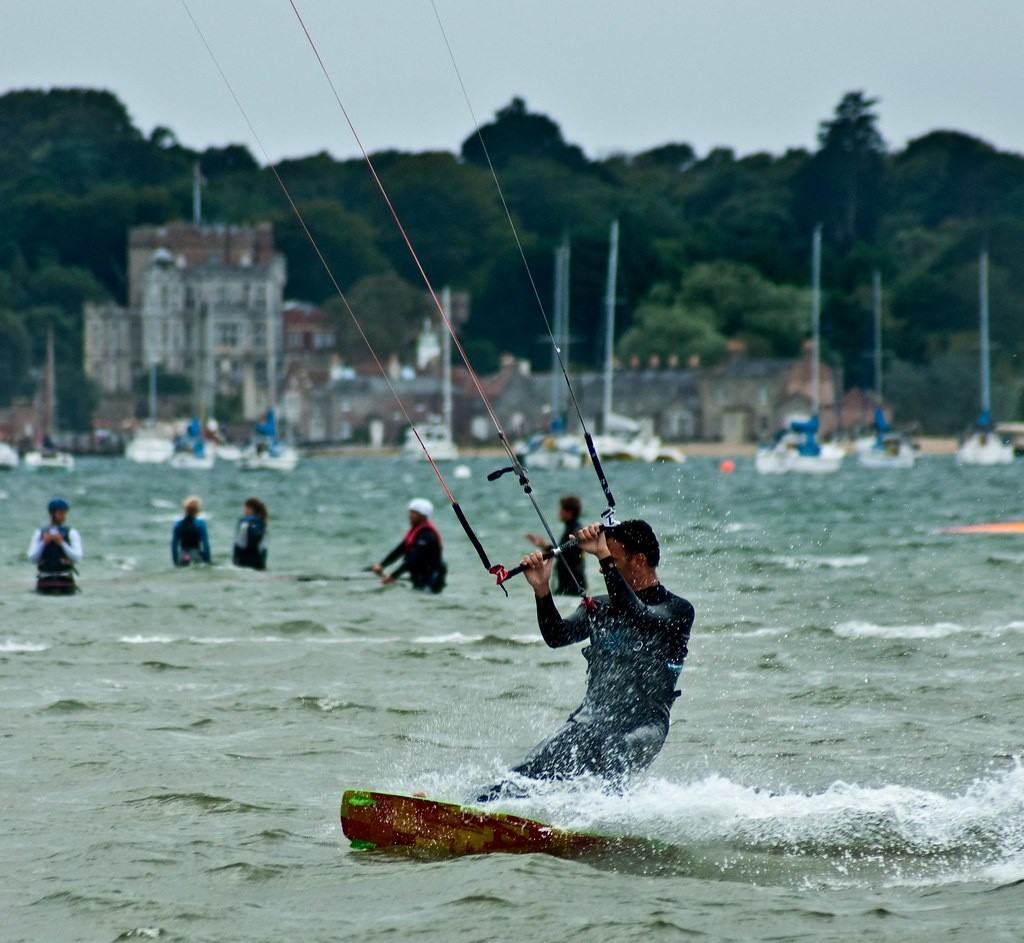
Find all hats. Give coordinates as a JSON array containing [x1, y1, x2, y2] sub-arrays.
[[408, 498, 433, 516], [48, 497, 68, 511]]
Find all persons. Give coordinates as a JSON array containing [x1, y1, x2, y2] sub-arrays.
[[477, 520, 696, 802], [233, 498, 267, 570], [27, 499, 82, 596], [527, 497, 587, 597], [170, 496, 212, 568], [373, 498, 448, 595]]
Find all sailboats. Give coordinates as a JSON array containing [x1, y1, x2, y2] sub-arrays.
[[755, 222, 846, 474], [849, 267, 919, 469], [514, 220, 684, 468], [124, 279, 302, 476], [402, 284, 463, 464], [950, 251, 1015, 468]]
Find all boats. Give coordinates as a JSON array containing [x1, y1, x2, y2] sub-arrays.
[[24, 434, 78, 474], [338, 788, 1022, 890]]
